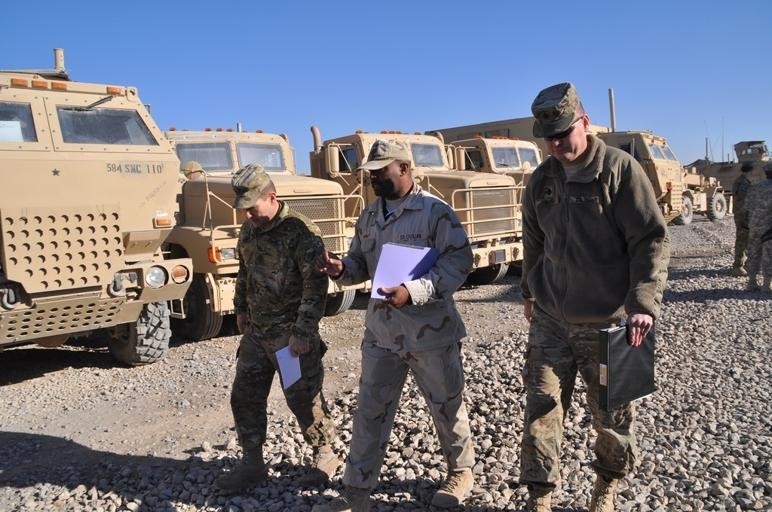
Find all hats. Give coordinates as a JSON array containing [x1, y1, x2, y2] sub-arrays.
[[531, 81, 580, 139], [231, 162, 271, 210], [357, 139, 414, 171]]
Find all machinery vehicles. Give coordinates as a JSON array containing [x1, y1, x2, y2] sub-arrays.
[[302, 126, 527, 284], [678, 166, 728, 226], [0, 50, 193, 370], [452, 133, 542, 193], [599, 130, 683, 224], [164, 130, 365, 338]]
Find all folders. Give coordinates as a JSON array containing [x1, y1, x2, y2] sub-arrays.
[[370, 241, 440, 299], [273, 346, 302, 391], [598, 322, 655, 413]]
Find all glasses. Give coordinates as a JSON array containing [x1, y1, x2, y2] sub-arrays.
[[543, 115, 584, 141]]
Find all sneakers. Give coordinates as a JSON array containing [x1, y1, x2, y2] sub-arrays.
[[300, 445, 340, 489]]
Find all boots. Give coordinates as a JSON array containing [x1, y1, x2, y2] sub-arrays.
[[748, 275, 759, 292], [215, 446, 269, 490], [759, 278, 772, 295], [524, 483, 554, 512], [732, 261, 748, 276], [589, 474, 619, 512], [310, 486, 373, 512], [431, 467, 475, 509]]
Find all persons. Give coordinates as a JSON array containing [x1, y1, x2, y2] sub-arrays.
[[742, 163, 772, 292], [732, 161, 756, 277], [311, 139, 476, 512], [519, 82, 671, 512], [216, 162, 346, 489]]
